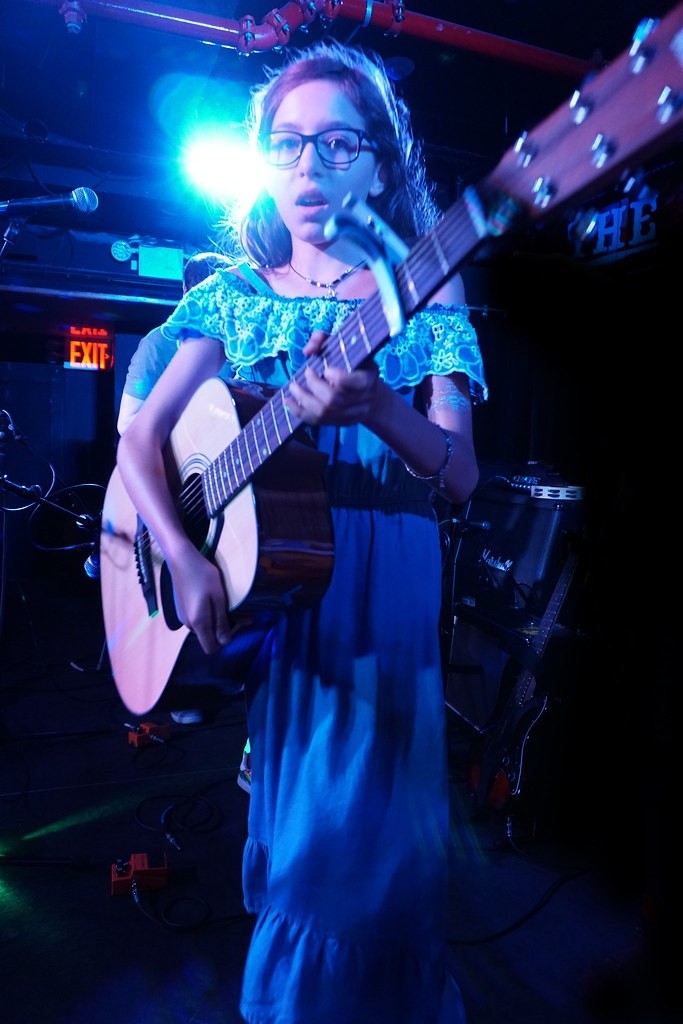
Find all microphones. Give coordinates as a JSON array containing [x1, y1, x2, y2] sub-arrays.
[[0, 187, 100, 214], [84, 513, 103, 580], [453, 517, 490, 533]]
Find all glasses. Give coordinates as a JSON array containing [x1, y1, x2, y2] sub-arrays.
[[259, 127, 385, 166]]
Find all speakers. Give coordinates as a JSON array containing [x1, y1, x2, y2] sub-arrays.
[[443, 602, 546, 751], [453, 484, 585, 649]]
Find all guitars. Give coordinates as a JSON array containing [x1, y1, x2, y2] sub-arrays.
[[466, 527, 594, 818], [95, 8, 682, 732]]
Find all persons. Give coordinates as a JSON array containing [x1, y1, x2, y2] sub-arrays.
[[117, 48, 488, 1024]]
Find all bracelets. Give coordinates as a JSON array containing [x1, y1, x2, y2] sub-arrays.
[[405, 424, 454, 488]]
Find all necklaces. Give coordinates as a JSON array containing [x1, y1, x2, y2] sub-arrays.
[[288, 259, 368, 300]]
